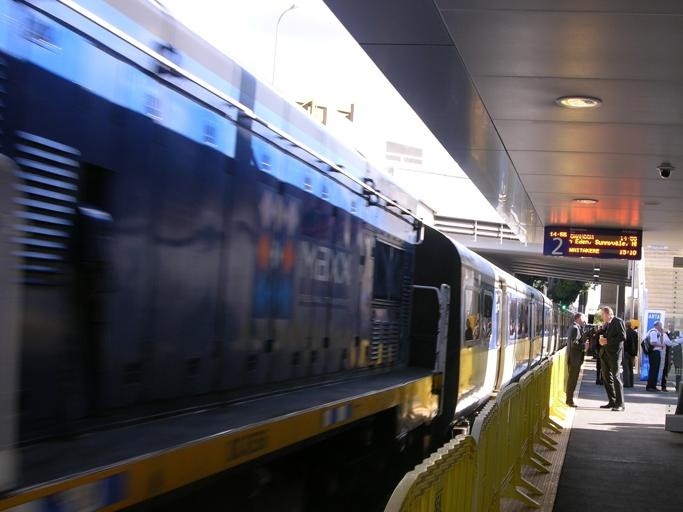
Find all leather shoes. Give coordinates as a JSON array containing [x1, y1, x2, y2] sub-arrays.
[[600, 404, 614, 408], [566, 400, 577, 407], [612, 405, 625, 411]]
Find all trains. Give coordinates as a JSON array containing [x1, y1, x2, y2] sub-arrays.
[[0, 0, 592, 512]]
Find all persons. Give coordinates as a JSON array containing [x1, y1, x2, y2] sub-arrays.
[[564, 306, 682, 412]]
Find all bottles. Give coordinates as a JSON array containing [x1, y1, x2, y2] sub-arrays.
[[599, 334, 605, 346]]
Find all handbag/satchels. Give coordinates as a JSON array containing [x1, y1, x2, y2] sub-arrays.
[[641, 336, 653, 354]]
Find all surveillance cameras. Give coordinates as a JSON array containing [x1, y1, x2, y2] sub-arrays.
[[656, 165, 676, 178]]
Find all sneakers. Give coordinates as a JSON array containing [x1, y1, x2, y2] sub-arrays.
[[645, 386, 669, 392]]
